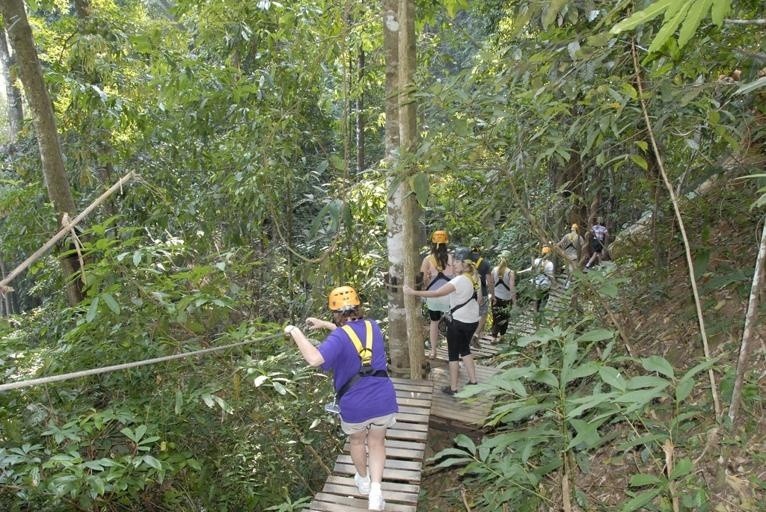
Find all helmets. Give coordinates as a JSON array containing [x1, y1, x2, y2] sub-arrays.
[[329, 286, 361, 311], [542, 247, 552, 257], [453, 247, 473, 259], [571, 223, 580, 231], [431, 230, 448, 245], [470, 237, 482, 248]]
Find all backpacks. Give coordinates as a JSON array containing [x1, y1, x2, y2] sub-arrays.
[[530, 274, 552, 294]]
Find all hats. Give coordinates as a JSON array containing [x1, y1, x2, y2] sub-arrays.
[[496, 249, 512, 257]]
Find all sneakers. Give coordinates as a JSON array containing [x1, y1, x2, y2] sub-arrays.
[[354, 472, 370, 495], [465, 379, 477, 385], [471, 335, 481, 349], [368, 493, 386, 511], [441, 386, 457, 395]]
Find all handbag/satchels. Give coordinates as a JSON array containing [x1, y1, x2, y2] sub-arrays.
[[438, 310, 453, 337]]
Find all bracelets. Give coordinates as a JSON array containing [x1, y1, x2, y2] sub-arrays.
[[289, 327, 295, 332]]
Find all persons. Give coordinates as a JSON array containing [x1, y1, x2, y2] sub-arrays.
[[406, 217, 609, 396], [283, 286, 397, 512]]
